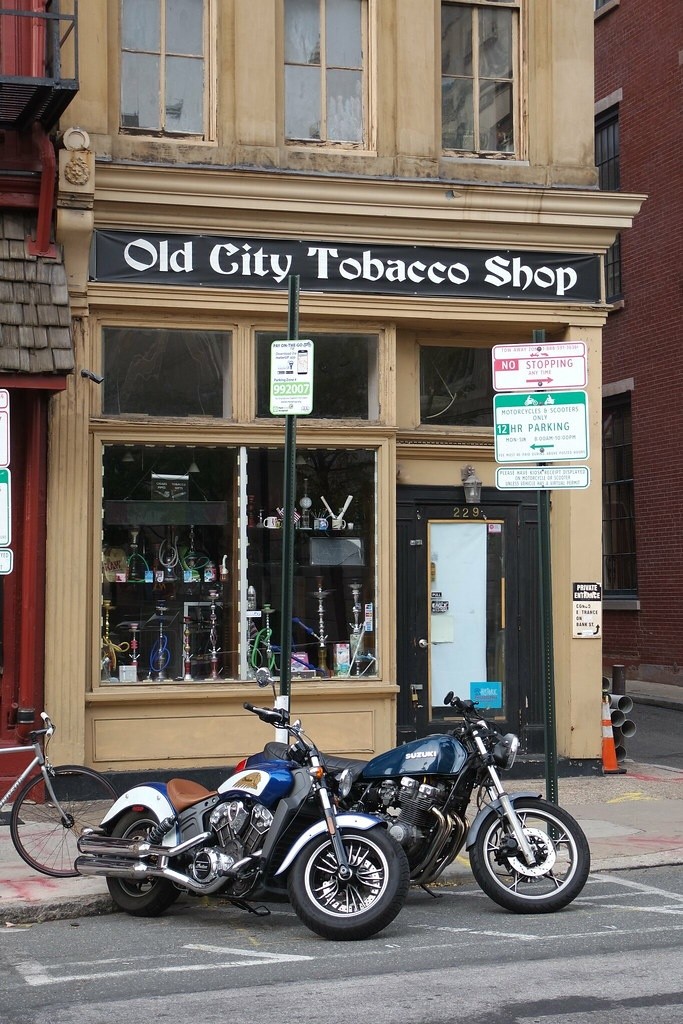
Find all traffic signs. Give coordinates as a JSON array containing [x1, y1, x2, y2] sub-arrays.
[[493, 391, 592, 465], [491, 340, 588, 392]]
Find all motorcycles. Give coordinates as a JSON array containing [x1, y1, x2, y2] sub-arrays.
[[73, 702, 412, 941], [235, 690, 591, 915]]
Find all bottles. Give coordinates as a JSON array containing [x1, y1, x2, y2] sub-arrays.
[[165, 559, 175, 581], [204, 565, 213, 582]]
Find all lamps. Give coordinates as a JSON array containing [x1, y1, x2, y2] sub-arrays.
[[461, 464, 482, 503]]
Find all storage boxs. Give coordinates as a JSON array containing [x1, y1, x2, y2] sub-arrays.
[[150, 473, 190, 501]]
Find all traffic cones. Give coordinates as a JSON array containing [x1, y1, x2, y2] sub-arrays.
[[603, 695, 628, 774]]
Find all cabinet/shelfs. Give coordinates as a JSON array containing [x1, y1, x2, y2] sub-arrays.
[[100, 497, 232, 680]]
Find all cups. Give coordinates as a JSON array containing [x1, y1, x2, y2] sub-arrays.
[[314, 518, 328, 530], [262, 516, 278, 528], [145, 571, 153, 583], [332, 519, 346, 530], [348, 523, 354, 529], [183, 571, 192, 583], [116, 573, 126, 582], [156, 571, 164, 582]]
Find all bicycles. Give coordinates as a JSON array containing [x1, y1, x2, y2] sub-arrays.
[[0, 711, 119, 879]]
